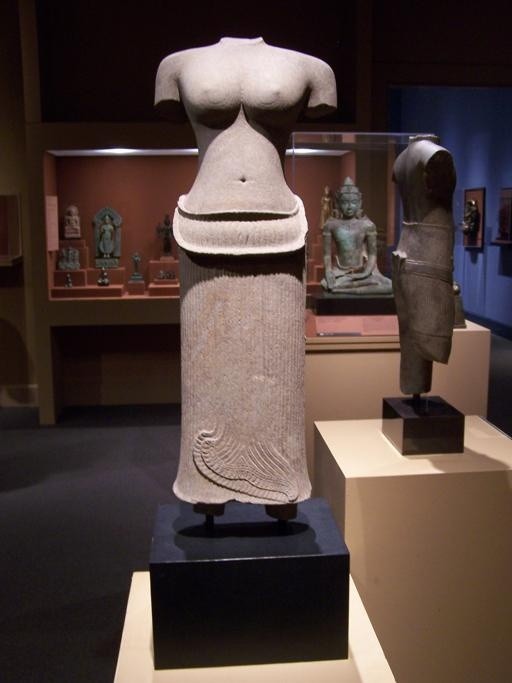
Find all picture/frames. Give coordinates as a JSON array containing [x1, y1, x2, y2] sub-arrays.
[[461, 185, 486, 251]]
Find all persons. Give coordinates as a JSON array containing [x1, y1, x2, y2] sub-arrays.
[[58, 206, 179, 285], [149, 34, 342, 535], [318, 179, 335, 231], [385, 133, 458, 396], [319, 176, 393, 295], [461, 198, 481, 240]]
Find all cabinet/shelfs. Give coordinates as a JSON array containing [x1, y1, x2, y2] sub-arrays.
[[302, 318, 492, 477], [47, 153, 352, 423], [312, 411, 512, 682], [109, 566, 401, 683]]
